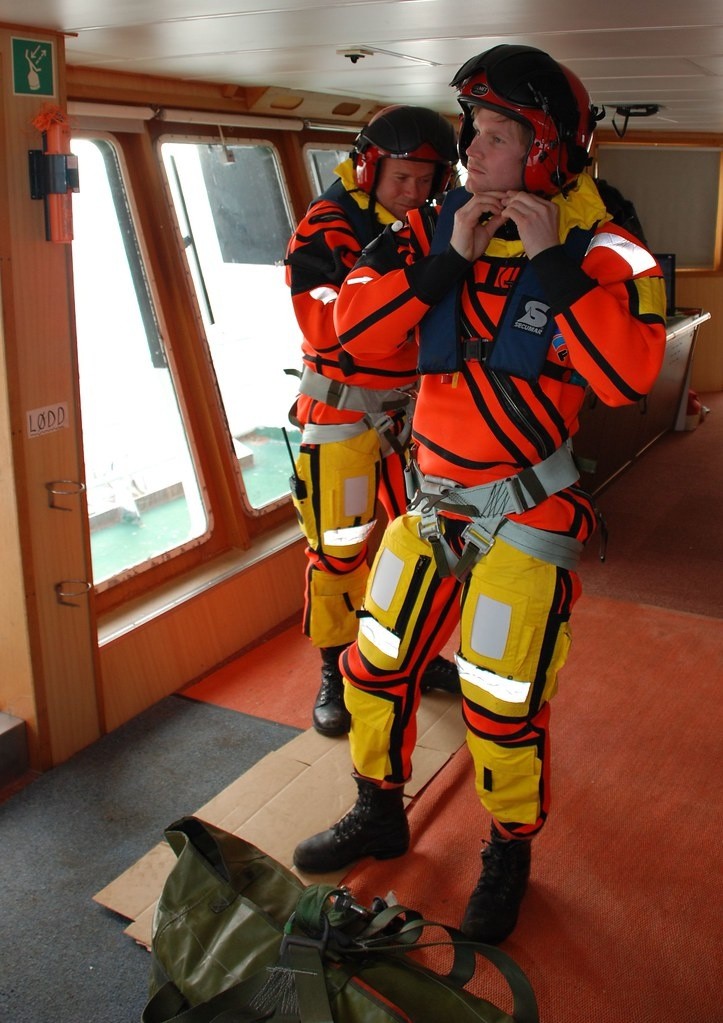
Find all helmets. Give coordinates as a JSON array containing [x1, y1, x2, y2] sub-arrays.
[[348, 105, 461, 212], [450, 44, 598, 198]]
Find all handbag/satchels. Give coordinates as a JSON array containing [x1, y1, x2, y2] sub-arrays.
[[139, 816, 538, 1023]]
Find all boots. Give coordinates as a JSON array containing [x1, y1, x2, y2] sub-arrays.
[[293, 772, 410, 875], [418, 654, 461, 697], [313, 643, 353, 739], [459, 819, 532, 947]]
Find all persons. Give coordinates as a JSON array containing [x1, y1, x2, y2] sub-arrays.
[[282, 105, 462, 739], [292, 44, 667, 939]]
